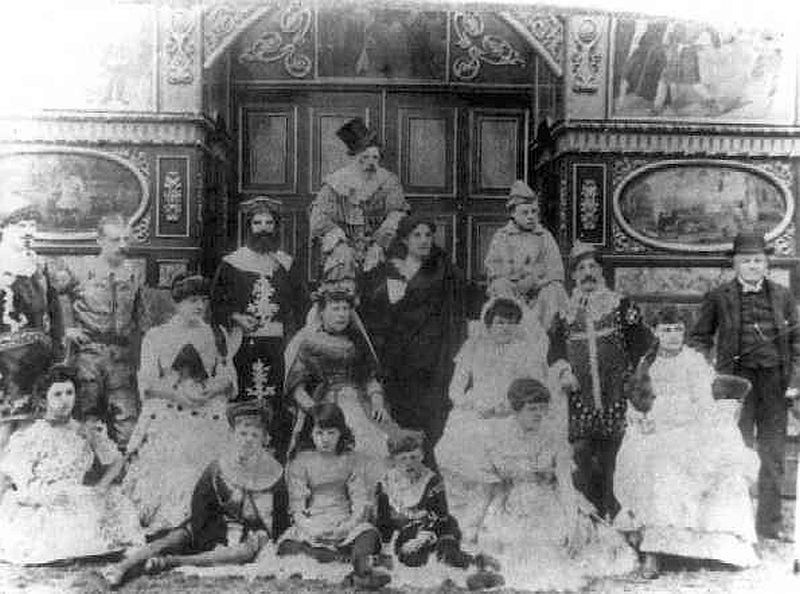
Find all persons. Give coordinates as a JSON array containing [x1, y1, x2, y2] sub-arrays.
[[0, 363, 143, 567], [434, 299, 568, 533], [309, 118, 411, 282], [685, 233, 800, 544], [483, 181, 570, 360], [612, 306, 761, 580], [545, 245, 660, 521], [283, 282, 399, 452], [459, 376, 640, 594], [622, 18, 721, 116], [101, 402, 288, 587], [208, 197, 312, 403], [275, 404, 391, 590], [121, 274, 239, 539], [373, 430, 505, 594], [359, 214, 489, 447], [66, 213, 151, 453], [0, 191, 68, 434]]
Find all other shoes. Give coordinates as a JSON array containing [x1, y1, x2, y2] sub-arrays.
[[141, 555, 172, 575], [466, 571, 505, 592], [640, 554, 659, 581], [343, 571, 391, 590], [100, 564, 125, 590], [437, 538, 471, 566]]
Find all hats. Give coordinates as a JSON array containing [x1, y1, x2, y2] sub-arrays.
[[338, 116, 379, 155], [507, 180, 537, 214], [225, 399, 272, 428], [386, 428, 425, 452], [725, 232, 774, 259]]
